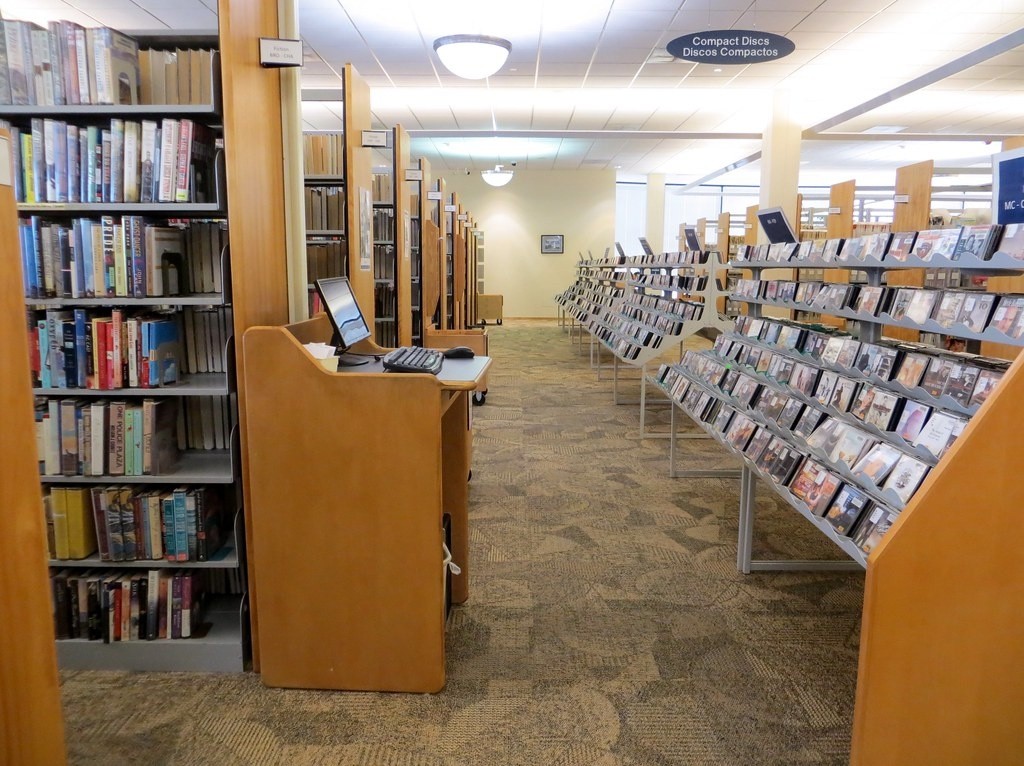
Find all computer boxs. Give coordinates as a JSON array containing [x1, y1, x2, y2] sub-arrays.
[[442, 512, 453, 620]]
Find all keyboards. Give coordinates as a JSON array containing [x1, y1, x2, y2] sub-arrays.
[[383, 346, 444, 375]]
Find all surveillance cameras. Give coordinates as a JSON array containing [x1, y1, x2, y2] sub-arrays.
[[512, 161, 516, 166], [465, 169, 470, 175]]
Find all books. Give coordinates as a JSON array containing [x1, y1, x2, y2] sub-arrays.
[[38, 480, 242, 563], [48, 562, 245, 644], [0, 9, 213, 105], [17, 215, 230, 298], [0, 118, 219, 205], [33, 392, 238, 477], [24, 303, 233, 390], [552, 217, 1024, 557], [301, 133, 452, 350]]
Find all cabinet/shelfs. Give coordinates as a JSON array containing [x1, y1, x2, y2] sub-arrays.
[[0, 0, 492, 766], [680, 136, 1023, 360]]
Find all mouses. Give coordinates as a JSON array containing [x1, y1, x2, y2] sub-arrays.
[[443, 346, 475, 358]]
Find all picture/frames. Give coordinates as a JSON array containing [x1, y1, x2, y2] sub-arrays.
[[541, 235, 563, 253]]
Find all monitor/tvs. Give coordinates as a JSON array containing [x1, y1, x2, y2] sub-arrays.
[[314, 276, 372, 367]]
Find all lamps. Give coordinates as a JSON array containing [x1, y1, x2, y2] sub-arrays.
[[480, 169, 514, 187], [432, 35, 511, 82]]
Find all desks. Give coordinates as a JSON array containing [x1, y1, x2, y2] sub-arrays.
[[241, 319, 470, 696]]
